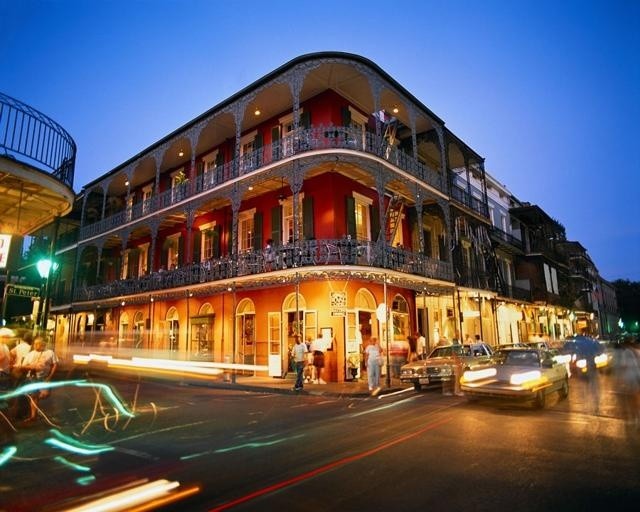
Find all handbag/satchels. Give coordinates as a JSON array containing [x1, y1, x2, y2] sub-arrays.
[[375, 347, 384, 367]]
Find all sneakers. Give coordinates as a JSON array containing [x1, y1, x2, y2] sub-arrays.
[[294, 377, 328, 391], [368, 386, 382, 391]]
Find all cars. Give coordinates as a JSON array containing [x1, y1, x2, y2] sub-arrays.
[[399, 334, 607, 409]]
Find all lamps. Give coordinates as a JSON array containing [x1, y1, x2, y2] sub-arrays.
[[277, 176, 287, 206]]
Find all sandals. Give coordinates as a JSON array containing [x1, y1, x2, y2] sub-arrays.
[[22, 415, 38, 423]]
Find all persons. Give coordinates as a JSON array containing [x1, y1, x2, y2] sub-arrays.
[[264, 238, 282, 269], [309, 331, 337, 385], [390, 336, 403, 379], [463, 333, 473, 343], [338, 233, 360, 264], [406, 335, 417, 365], [0, 325, 60, 428], [611, 333, 640, 422], [559, 325, 606, 416], [415, 331, 427, 360], [290, 333, 308, 390], [475, 334, 481, 343], [365, 337, 386, 391]]
